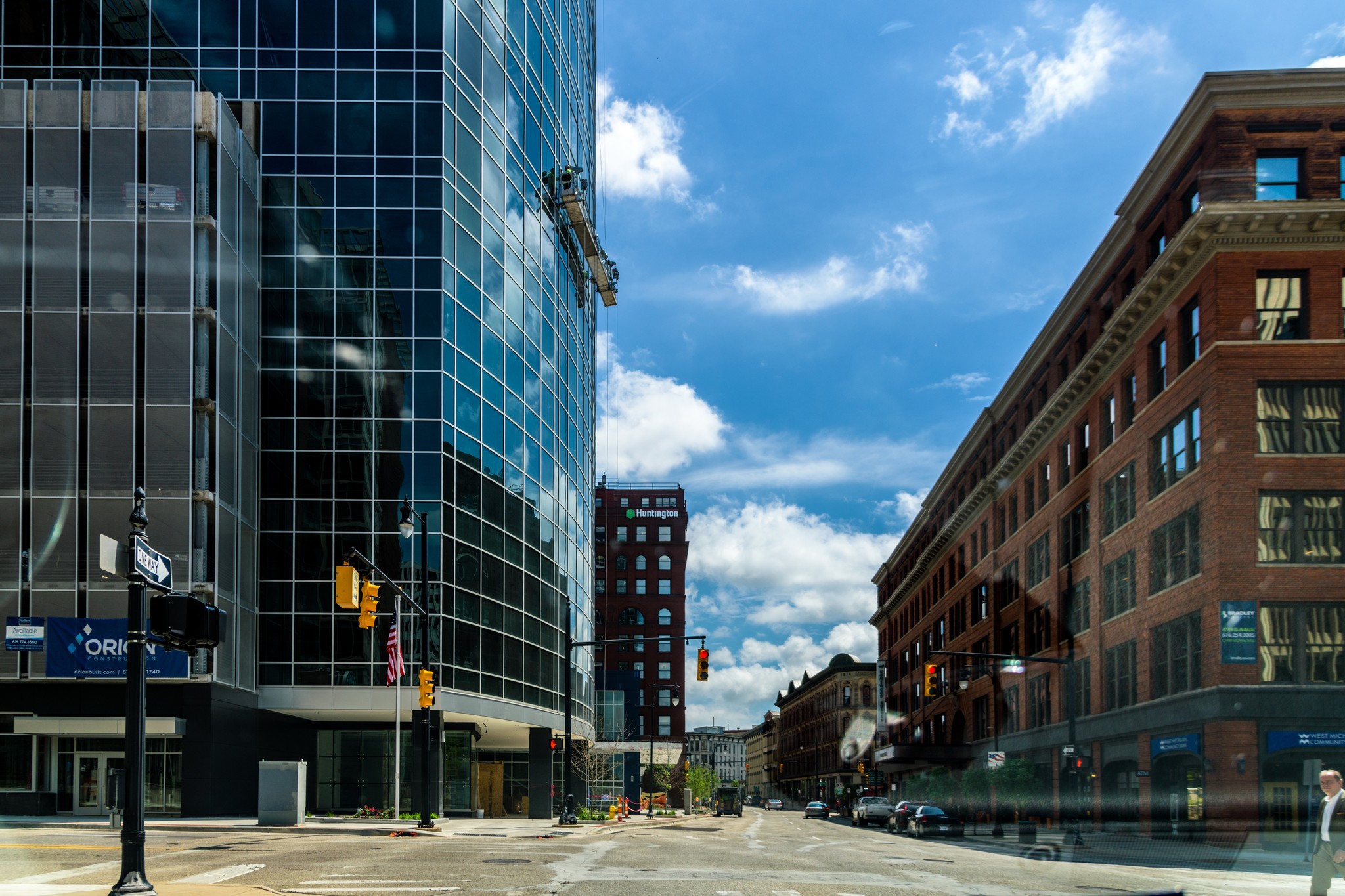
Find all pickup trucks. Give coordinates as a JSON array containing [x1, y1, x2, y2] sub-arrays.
[[849, 796, 896, 828]]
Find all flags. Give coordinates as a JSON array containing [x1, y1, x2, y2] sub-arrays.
[[387, 599, 404, 687]]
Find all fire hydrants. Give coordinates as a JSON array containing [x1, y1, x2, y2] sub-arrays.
[[608, 804, 618, 820]]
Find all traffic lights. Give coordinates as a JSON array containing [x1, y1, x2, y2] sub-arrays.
[[358, 581, 382, 629], [165, 592, 209, 645], [1077, 755, 1091, 767], [697, 648, 708, 681], [924, 664, 937, 696], [549, 737, 563, 751], [746, 764, 749, 772], [418, 669, 435, 708], [780, 763, 784, 773]]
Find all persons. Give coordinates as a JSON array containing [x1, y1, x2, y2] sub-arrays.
[[1309, 770, 1345, 896], [835, 800, 839, 814]]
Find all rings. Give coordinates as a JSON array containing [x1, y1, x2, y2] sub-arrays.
[[1339, 857, 1342, 859]]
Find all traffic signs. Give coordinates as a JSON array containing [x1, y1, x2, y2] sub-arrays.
[[135, 534, 173, 590], [1061, 745, 1075, 755]]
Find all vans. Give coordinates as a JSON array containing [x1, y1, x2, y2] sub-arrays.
[[715, 787, 743, 818]]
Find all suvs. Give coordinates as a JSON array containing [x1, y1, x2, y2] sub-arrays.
[[887, 800, 931, 834]]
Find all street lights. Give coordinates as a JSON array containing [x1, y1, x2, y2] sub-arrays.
[[626, 682, 680, 820], [397, 492, 443, 833], [684, 732, 690, 788], [959, 659, 1005, 838], [762, 763, 772, 799], [712, 742, 728, 789]]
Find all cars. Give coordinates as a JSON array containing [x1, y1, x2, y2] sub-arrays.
[[742, 795, 762, 806], [805, 800, 830, 819], [759, 797, 772, 807], [765, 799, 782, 810], [906, 804, 964, 841]]
